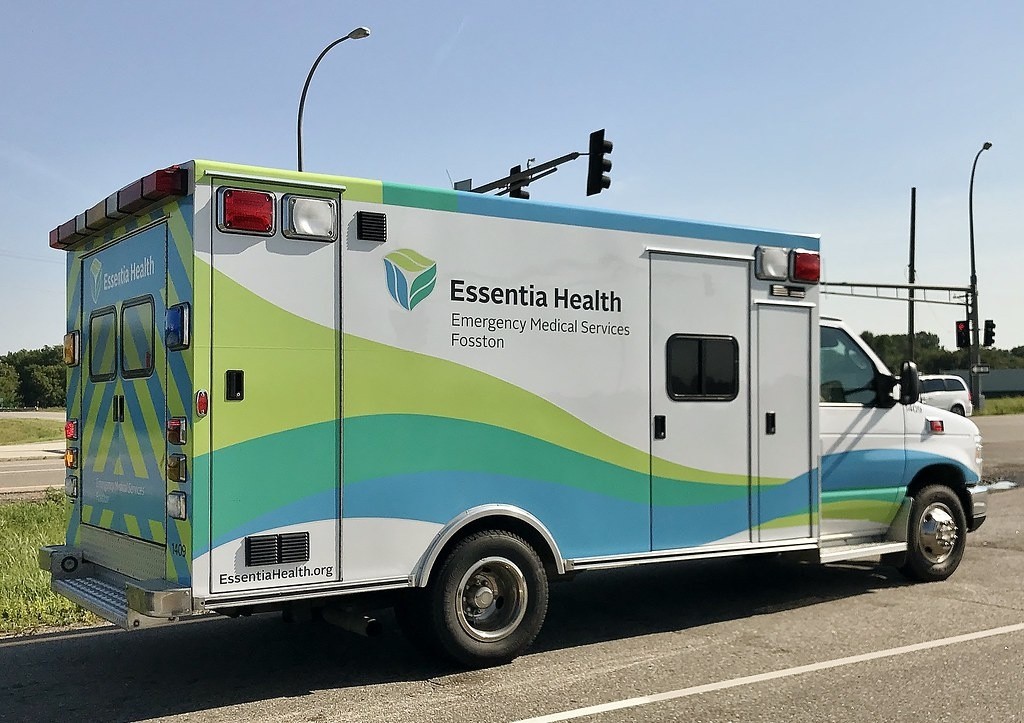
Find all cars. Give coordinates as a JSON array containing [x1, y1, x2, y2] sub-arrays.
[[889, 374, 973, 418]]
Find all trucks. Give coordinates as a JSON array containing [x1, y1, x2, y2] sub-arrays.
[[35, 156, 989, 665]]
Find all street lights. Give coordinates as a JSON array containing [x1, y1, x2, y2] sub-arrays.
[[296, 26, 371, 172], [969, 141, 992, 410]]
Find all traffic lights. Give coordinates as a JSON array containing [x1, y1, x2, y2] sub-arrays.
[[983, 320, 996, 347], [955, 320, 969, 348], [586, 129, 613, 197], [509, 164, 532, 200]]
